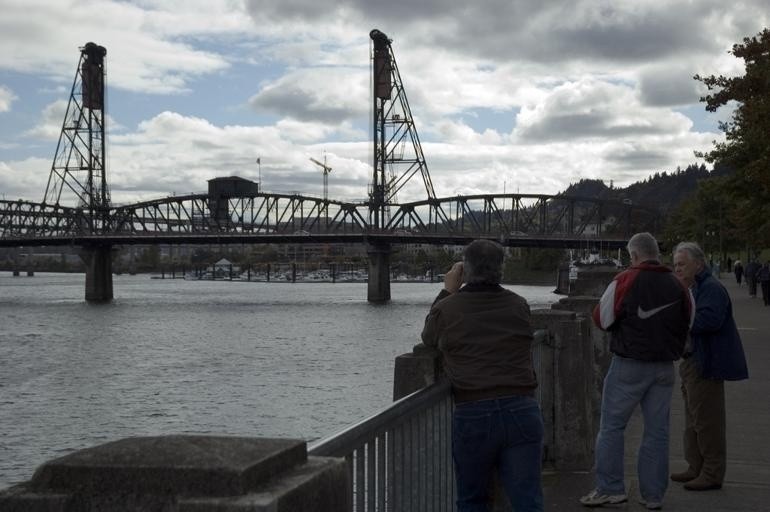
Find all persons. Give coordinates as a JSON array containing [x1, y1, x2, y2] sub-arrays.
[[727, 257, 770, 306], [670, 241, 748, 492], [421, 238, 547, 512], [578, 231, 696, 509]]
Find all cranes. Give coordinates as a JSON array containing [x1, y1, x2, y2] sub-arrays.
[[309, 148, 333, 234]]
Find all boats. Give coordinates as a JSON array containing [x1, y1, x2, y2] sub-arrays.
[[567, 237, 626, 296], [240, 268, 441, 284]]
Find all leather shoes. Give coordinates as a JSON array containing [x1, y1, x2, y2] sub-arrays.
[[685, 473, 723, 490], [672, 465, 699, 482]]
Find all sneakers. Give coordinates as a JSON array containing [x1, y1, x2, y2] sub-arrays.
[[580, 489, 629, 506], [640, 496, 663, 509]]
